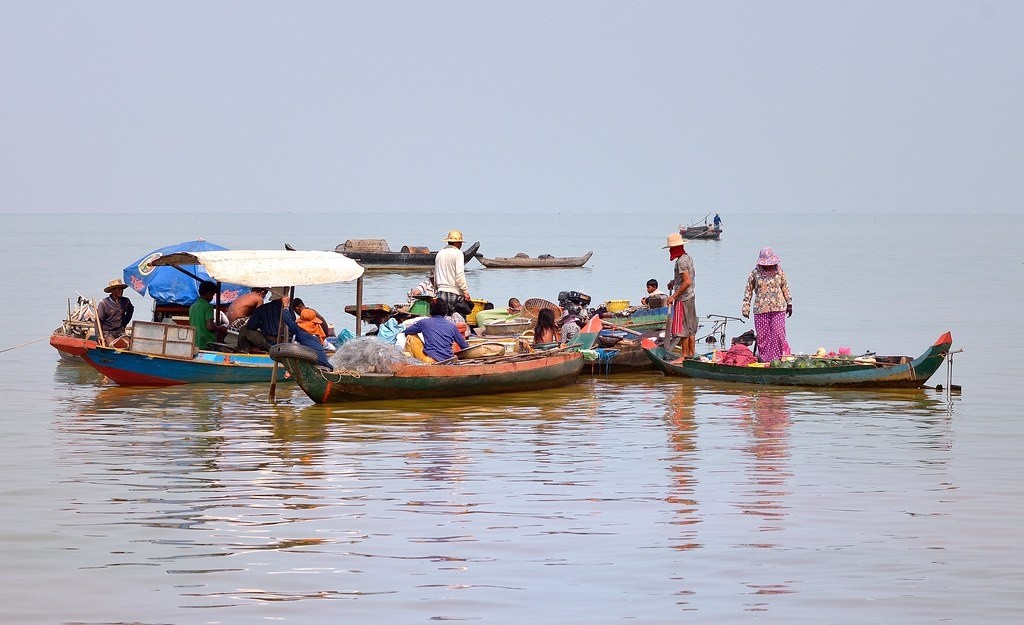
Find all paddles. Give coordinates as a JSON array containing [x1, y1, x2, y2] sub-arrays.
[[663, 280, 674, 349], [268, 286, 290, 404], [690, 212, 711, 227], [91, 298, 110, 383], [690, 224, 723, 240]]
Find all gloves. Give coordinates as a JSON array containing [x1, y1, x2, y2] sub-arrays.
[[786, 304, 792, 318]]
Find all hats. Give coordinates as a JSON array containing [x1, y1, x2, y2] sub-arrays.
[[295, 308, 322, 324], [442, 230, 466, 243], [104, 279, 128, 293], [426, 267, 435, 278], [269, 287, 284, 300], [757, 247, 780, 266], [662, 233, 689, 249]]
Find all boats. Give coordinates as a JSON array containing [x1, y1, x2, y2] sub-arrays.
[[639, 331, 952, 386], [477, 251, 594, 268], [680, 225, 722, 238], [51, 239, 365, 389], [284, 239, 480, 274], [271, 294, 674, 404]]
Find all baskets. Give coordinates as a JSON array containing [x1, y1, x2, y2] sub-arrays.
[[603, 299, 631, 312], [522, 298, 563, 326]]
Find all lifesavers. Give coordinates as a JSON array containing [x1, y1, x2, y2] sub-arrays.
[[269, 343, 318, 364]]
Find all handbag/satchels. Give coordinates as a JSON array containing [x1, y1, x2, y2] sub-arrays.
[[450, 296, 474, 315]]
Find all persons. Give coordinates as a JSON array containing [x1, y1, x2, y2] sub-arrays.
[[641, 279, 668, 308], [742, 248, 793, 364], [96, 279, 134, 347], [534, 308, 560, 345], [409, 269, 439, 315], [226, 286, 333, 380], [188, 280, 228, 352], [713, 213, 723, 235], [75, 296, 93, 318], [365, 298, 469, 365], [558, 310, 584, 348], [667, 233, 696, 365], [507, 298, 524, 316], [434, 230, 471, 329]]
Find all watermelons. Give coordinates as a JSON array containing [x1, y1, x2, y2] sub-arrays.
[[769, 353, 856, 369]]
